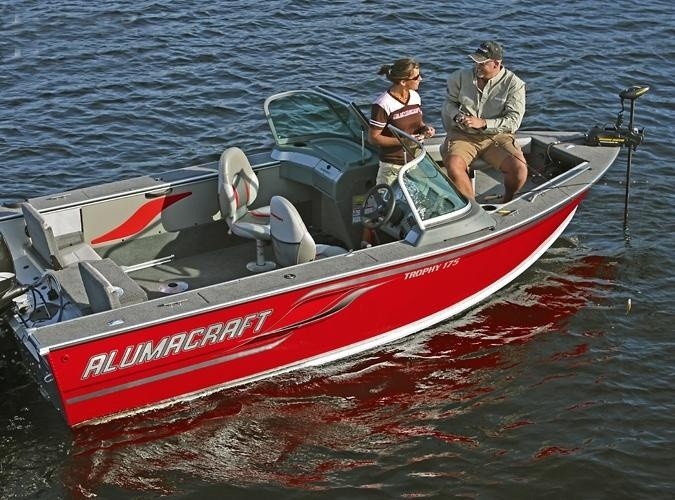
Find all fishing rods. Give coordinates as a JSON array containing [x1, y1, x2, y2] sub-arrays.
[[454, 101, 650, 214]]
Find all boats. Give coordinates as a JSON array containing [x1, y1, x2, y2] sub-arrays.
[[0, 88, 621, 429]]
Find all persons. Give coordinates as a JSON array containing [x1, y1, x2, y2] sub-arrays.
[[441, 39, 527, 203], [359, 58, 435, 248]]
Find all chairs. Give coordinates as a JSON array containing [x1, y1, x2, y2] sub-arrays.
[[217, 147, 346, 270], [20, 201, 147, 316]]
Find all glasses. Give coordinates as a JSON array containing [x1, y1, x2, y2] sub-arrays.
[[407, 74, 420, 80]]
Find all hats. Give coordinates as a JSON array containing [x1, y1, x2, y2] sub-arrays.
[[468, 40, 504, 64]]
